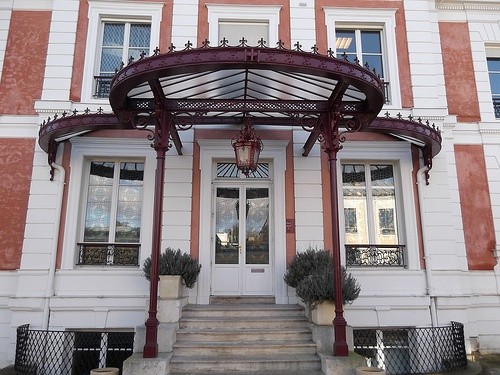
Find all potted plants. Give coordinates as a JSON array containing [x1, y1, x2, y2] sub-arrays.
[[296, 265, 361, 326], [143, 247, 201, 299], [282, 249, 334, 319]]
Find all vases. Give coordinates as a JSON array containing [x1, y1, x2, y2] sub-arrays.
[[355, 367, 385, 375], [90, 367, 120, 375]]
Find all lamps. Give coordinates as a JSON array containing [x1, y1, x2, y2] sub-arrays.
[[231, 117, 264, 177]]
